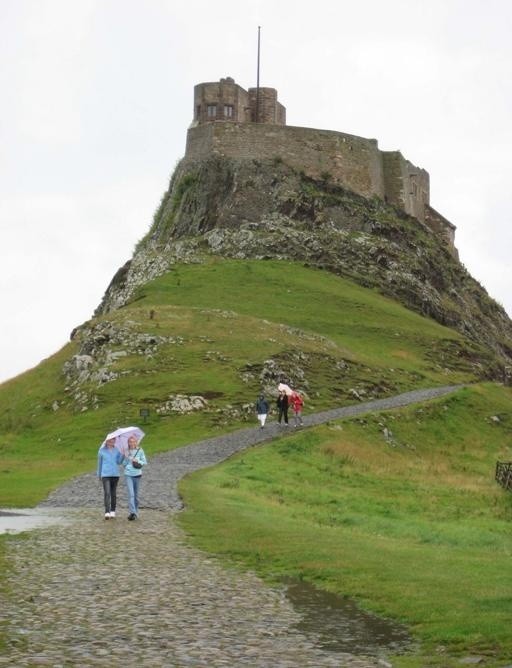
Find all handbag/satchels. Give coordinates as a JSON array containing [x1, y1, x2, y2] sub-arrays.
[[133, 462, 142, 469]]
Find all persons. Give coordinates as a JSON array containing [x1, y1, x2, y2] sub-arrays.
[[287, 391, 304, 427], [97, 438, 125, 520], [256, 393, 269, 429], [122, 436, 148, 520], [276, 390, 289, 426]]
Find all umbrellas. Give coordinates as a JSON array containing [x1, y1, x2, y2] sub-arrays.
[[278, 383, 292, 396], [100, 426, 146, 452]]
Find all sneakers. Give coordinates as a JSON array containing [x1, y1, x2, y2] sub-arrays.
[[261, 422, 304, 428], [128, 513, 137, 521], [104, 512, 116, 519]]
